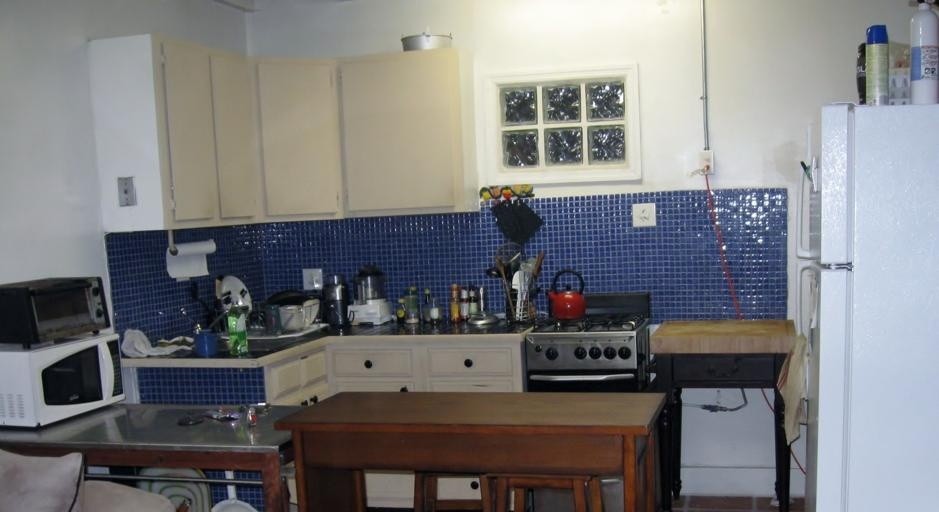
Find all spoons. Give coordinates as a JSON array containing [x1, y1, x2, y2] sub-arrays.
[[488, 267, 501, 280]]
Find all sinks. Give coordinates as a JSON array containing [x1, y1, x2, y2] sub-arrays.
[[217, 337, 304, 352]]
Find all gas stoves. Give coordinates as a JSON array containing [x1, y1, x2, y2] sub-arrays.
[[525, 312, 652, 371]]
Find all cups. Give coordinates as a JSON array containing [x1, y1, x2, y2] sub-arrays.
[[195, 328, 220, 357], [264, 304, 282, 336]]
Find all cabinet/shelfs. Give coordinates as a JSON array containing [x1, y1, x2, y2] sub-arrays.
[[122, 338, 331, 512], [329, 334, 526, 512], [251, 57, 344, 221], [87, 34, 265, 234], [344, 48, 479, 216]]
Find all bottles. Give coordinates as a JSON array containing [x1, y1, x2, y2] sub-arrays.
[[405, 285, 420, 330], [451, 283, 460, 324], [460, 285, 470, 320], [228, 293, 247, 356], [856, 44, 870, 107], [469, 285, 478, 320], [431, 296, 438, 323], [480, 287, 488, 320], [866, 23, 891, 107], [423, 289, 433, 328], [397, 297, 405, 326], [911, 5, 939, 108]]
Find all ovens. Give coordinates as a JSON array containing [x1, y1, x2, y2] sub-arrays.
[[0, 273, 111, 352]]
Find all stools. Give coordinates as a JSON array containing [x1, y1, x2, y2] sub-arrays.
[[495, 473, 604, 512], [408, 471, 490, 512]]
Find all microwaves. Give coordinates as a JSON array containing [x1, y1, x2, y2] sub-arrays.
[[0, 333, 125, 431]]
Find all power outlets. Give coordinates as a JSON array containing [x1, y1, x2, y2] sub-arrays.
[[632, 203, 656, 228], [699, 150, 712, 176]]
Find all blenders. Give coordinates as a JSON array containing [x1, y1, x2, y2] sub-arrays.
[[347, 265, 392, 326]]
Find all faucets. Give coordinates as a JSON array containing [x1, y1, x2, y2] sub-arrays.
[[205, 303, 249, 331]]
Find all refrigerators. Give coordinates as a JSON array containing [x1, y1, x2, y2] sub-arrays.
[[793, 102, 939, 511]]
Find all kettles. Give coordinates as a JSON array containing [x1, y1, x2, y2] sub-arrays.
[[544, 267, 588, 320]]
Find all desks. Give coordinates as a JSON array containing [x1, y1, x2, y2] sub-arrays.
[[273, 390, 669, 512], [0, 403, 306, 512]]
